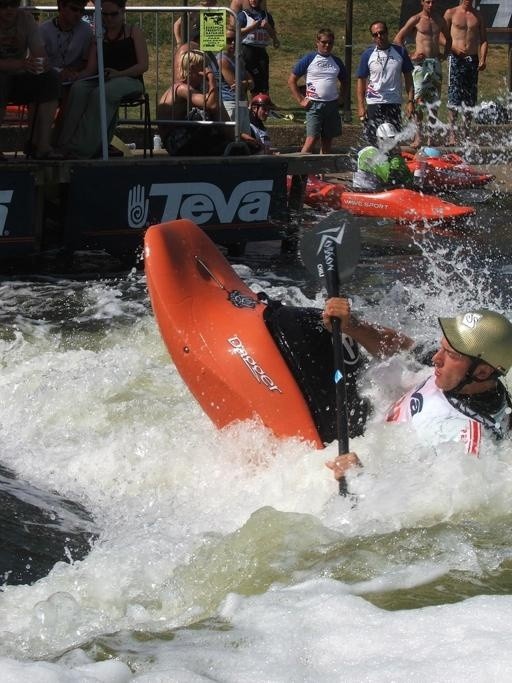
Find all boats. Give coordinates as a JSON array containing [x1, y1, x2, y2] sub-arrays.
[[399, 151, 496, 189], [287, 175, 475, 221], [144, 220, 379, 513]]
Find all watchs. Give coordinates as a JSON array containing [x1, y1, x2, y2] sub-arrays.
[[408, 99, 415, 103]]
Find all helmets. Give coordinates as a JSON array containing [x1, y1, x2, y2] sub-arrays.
[[251, 93, 276, 109], [376, 122, 398, 137], [437, 308, 512, 378]]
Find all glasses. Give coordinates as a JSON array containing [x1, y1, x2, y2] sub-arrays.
[[0, 0, 19, 8], [371, 30, 384, 36], [102, 8, 122, 15], [320, 40, 333, 44], [60, 4, 86, 15]]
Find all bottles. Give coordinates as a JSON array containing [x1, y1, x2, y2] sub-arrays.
[[153, 135, 161, 151]]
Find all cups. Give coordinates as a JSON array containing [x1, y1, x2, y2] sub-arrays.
[[34, 57, 44, 74]]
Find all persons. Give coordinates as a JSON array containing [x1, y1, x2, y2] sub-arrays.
[[352, 122, 413, 192], [288, 28, 346, 153], [36, 0, 94, 158], [393, 0, 453, 148], [157, 0, 280, 156], [323, 298, 512, 481], [0, 0, 64, 159], [356, 21, 415, 143], [55, 0, 149, 159], [439, 0, 488, 145]]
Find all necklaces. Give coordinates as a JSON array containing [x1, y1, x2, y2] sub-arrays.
[[104, 21, 124, 44]]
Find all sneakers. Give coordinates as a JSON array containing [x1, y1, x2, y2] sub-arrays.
[[31, 148, 64, 161]]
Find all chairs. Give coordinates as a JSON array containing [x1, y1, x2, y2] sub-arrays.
[[0, 99, 37, 160], [105, 74, 153, 159]]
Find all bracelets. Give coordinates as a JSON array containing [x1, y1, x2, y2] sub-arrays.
[[358, 114, 368, 122]]
